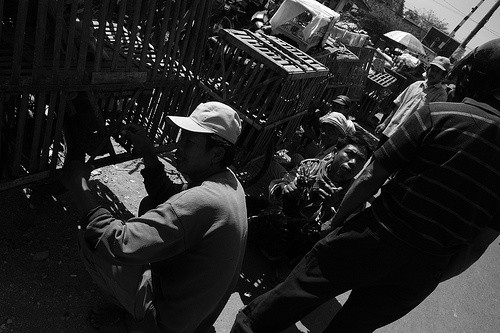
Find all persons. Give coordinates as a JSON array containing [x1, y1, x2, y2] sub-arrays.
[[65, 38, 500, 333]]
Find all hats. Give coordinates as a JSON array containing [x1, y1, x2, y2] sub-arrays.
[[167, 101, 242, 143], [430, 56, 450, 71], [321, 112, 348, 138], [332, 95, 351, 106]]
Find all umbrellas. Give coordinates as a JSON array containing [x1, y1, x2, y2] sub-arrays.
[[383, 30, 427, 63]]
